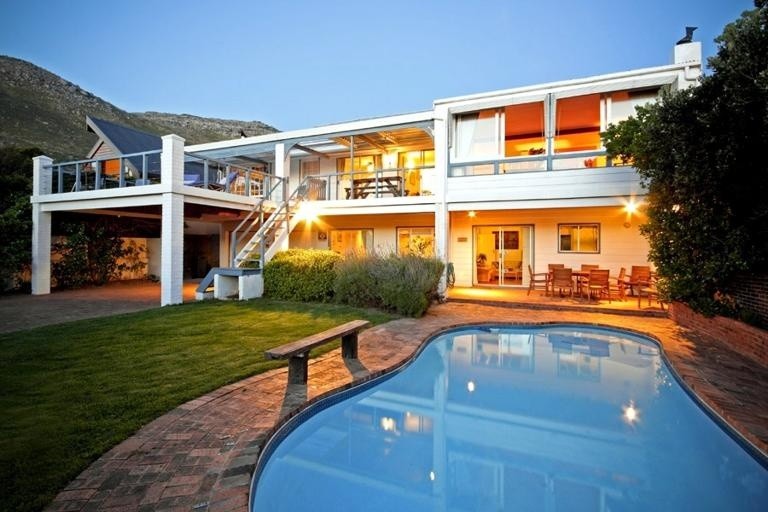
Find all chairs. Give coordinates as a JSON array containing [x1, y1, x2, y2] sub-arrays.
[[134, 169, 240, 192], [529, 329, 628, 358], [527, 263, 669, 313]]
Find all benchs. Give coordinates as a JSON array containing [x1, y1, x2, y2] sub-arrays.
[[344, 176, 406, 199], [264, 319, 372, 385]]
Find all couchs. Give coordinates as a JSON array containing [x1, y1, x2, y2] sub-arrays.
[[477, 260, 523, 283]]
